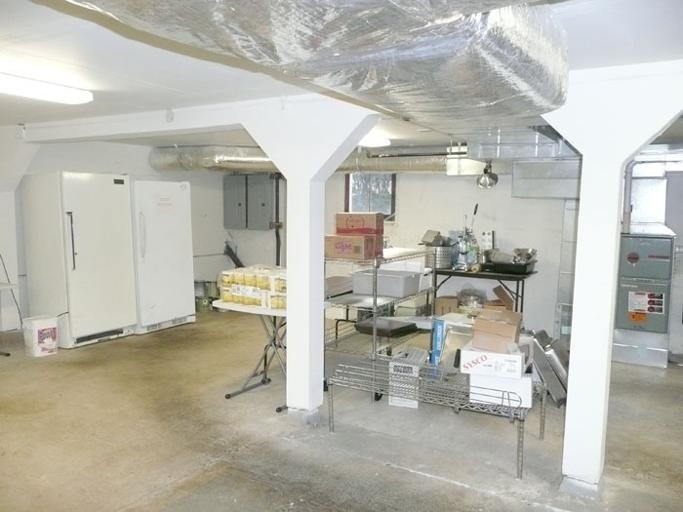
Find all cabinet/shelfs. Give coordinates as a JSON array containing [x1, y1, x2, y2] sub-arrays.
[[223, 174, 275, 231], [324, 251, 437, 402]]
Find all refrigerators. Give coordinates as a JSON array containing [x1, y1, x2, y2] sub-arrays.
[[22, 171, 196, 350]]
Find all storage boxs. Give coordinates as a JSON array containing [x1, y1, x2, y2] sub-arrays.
[[389, 286, 569, 410], [350, 269, 421, 298], [324, 212, 385, 266], [495, 259, 537, 274]]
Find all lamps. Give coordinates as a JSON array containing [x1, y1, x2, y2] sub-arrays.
[[477, 161, 498, 189]]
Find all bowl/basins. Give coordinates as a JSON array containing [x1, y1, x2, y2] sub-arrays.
[[513, 248, 537, 260]]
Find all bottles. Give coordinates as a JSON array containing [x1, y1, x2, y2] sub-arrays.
[[452, 234, 479, 270]]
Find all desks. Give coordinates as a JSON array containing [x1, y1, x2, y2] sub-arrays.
[[211, 299, 287, 412], [426, 265, 530, 317]]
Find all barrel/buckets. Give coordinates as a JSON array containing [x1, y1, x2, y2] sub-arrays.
[[21, 315, 59, 358], [197, 296, 210, 313], [425, 246, 453, 270]]
